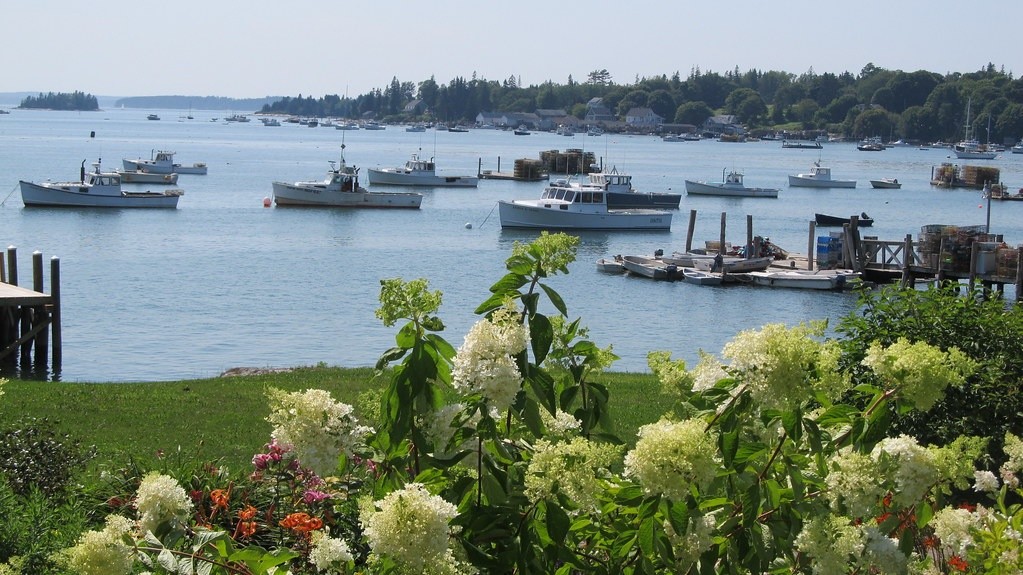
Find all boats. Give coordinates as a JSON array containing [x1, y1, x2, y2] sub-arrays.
[[782, 134, 845, 149], [660, 127, 784, 142], [209, 112, 386, 130], [548, 125, 574, 136], [147, 114, 160, 120], [787, 151, 857, 188], [929, 163, 1023, 201], [19, 148, 208, 209], [595, 253, 774, 286], [272, 86, 423, 208], [856, 134, 910, 151], [367, 131, 478, 187], [477, 148, 682, 232], [920, 98, 1023, 160], [684, 160, 783, 198], [815, 212, 875, 228], [870, 177, 902, 189], [495, 123, 530, 135], [587, 124, 603, 136], [405, 120, 469, 132]]
